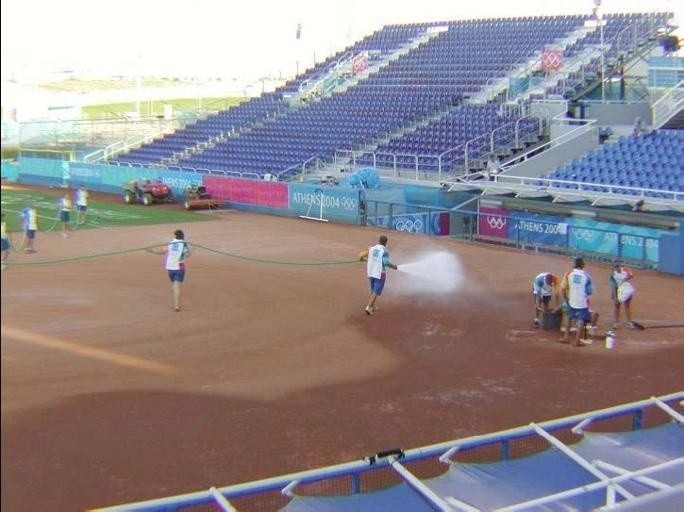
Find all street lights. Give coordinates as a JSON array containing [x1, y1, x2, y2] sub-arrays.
[[596, 18, 608, 103]]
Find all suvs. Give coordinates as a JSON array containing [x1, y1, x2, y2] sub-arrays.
[[121, 176, 176, 208]]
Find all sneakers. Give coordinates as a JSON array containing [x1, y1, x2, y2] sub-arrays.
[[611, 321, 637, 330], [365, 304, 378, 316], [175, 304, 182, 311], [532, 318, 597, 347]]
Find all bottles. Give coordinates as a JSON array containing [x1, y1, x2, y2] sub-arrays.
[[605, 330, 615, 349]]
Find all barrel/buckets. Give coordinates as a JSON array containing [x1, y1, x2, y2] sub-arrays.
[[544, 308, 563, 331]]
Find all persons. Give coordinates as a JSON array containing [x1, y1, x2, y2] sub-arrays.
[[58, 193, 72, 237], [533, 272, 560, 328], [0, 214, 12, 267], [632, 117, 644, 139], [20, 200, 38, 254], [74, 185, 89, 224], [487, 153, 502, 180], [358, 235, 395, 316], [162, 229, 190, 311], [609, 262, 637, 329], [559, 258, 592, 346]]
